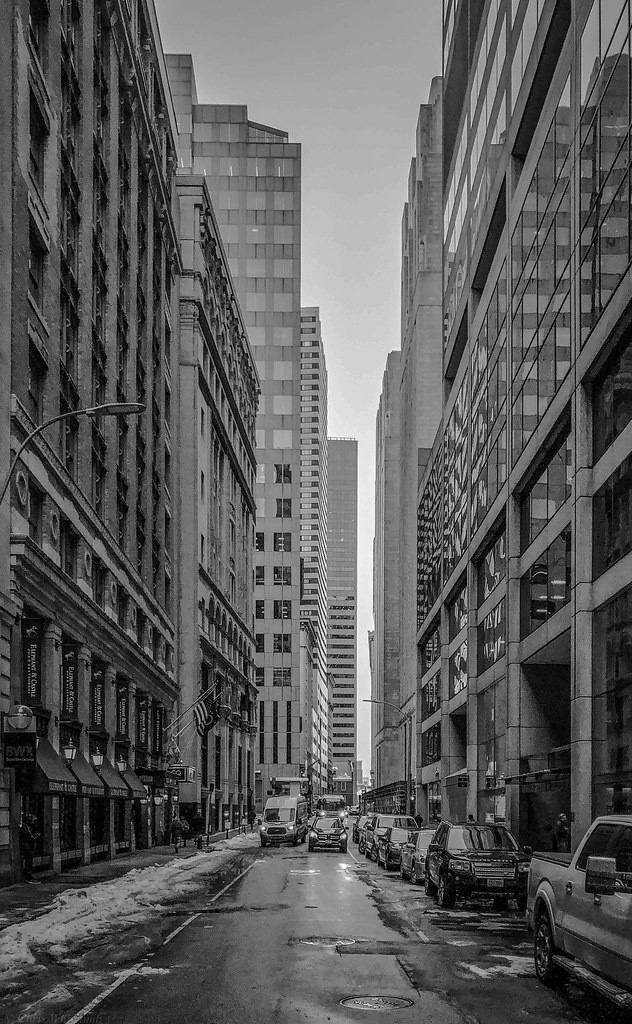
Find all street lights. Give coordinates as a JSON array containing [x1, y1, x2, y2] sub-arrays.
[[0, 402, 148, 502], [363, 699, 410, 726]]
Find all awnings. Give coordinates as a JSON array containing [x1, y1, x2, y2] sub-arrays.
[[15, 736, 147, 800], [500, 766, 571, 787], [444, 767, 466, 781]]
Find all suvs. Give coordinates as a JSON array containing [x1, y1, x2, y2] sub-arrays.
[[421, 820, 534, 912]]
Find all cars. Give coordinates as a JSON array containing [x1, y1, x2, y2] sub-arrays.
[[349, 805, 361, 816], [377, 827, 412, 871], [306, 816, 350, 854], [397, 829, 440, 885], [351, 815, 377, 844], [345, 810, 349, 818]]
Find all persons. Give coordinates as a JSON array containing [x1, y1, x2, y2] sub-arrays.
[[467, 814, 476, 823], [249, 810, 256, 830], [169, 818, 184, 853], [19, 814, 42, 885], [193, 811, 205, 845], [180, 817, 190, 847], [554, 813, 569, 853], [414, 813, 423, 828]]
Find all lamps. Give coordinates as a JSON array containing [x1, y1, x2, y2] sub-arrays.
[[172, 792, 179, 802], [153, 793, 164, 806], [139, 794, 150, 804], [161, 791, 168, 802], [90, 746, 103, 776], [62, 737, 77, 768], [116, 754, 127, 776]]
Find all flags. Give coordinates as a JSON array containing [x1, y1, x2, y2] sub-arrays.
[[192, 693, 213, 727], [195, 702, 221, 738]]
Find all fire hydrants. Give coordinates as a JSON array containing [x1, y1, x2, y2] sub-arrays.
[[197, 835, 204, 850]]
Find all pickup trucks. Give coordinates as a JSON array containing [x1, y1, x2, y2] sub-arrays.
[[523, 814, 632, 1004]]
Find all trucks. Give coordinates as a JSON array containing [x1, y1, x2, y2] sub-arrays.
[[314, 793, 346, 823]]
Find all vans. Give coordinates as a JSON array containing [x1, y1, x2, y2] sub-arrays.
[[359, 810, 420, 863], [257, 795, 309, 847]]
